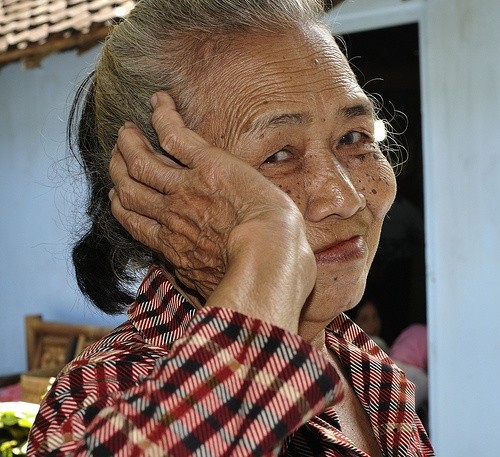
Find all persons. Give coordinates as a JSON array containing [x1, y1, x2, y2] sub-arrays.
[[28, 0, 437, 457]]
